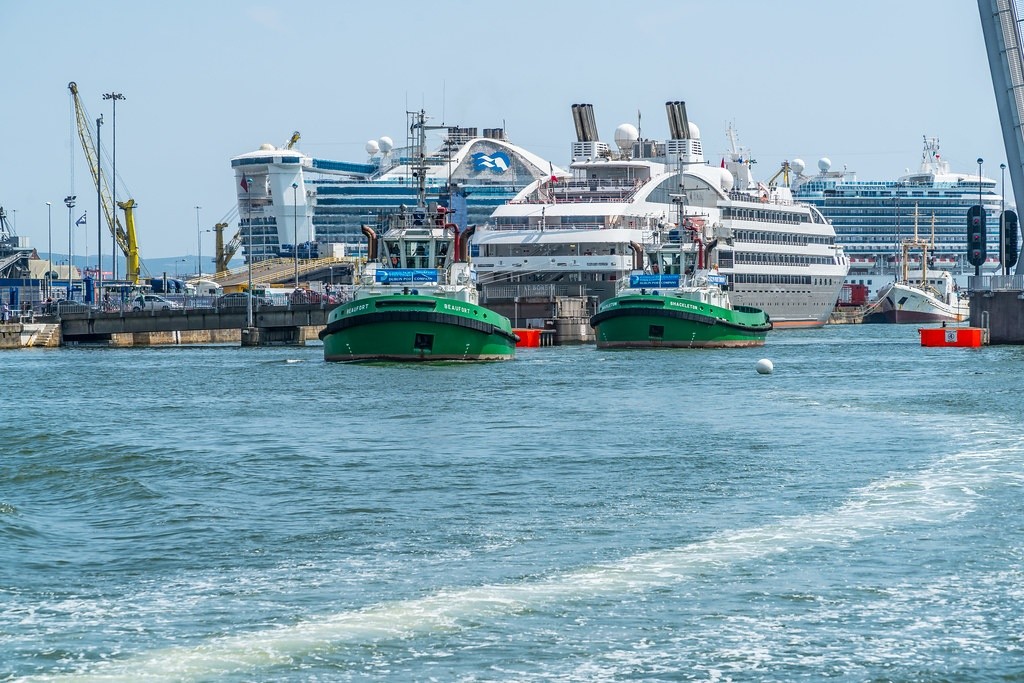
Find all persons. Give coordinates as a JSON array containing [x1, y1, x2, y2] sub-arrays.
[[325, 282, 332, 304], [21, 301, 27, 323], [0, 300, 9, 325], [140, 290, 146, 310], [25, 301, 31, 322], [390, 244, 400, 268], [103, 290, 115, 311], [760, 194, 768, 203], [46, 297, 53, 315], [440, 244, 447, 268], [413, 245, 426, 268]]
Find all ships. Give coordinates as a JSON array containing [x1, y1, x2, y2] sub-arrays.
[[470, 100, 852, 329], [231, 99, 566, 262], [228, 126, 567, 265], [791, 133, 1018, 275]]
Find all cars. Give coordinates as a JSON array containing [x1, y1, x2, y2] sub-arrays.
[[131, 294, 180, 311], [211, 291, 274, 307], [289, 289, 336, 302]]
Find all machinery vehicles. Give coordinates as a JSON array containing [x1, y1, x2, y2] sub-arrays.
[[210, 130, 302, 273], [65, 80, 149, 286]]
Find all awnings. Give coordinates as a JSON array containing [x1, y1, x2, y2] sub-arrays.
[[151, 278, 177, 289], [172, 279, 187, 290]]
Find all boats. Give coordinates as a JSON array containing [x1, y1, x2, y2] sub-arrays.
[[585, 188, 776, 349], [319, 108, 519, 360], [860, 200, 972, 324]]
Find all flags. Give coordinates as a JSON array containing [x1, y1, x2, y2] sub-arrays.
[[720, 157, 724, 168], [75, 213, 86, 227], [240, 173, 248, 192], [550, 165, 559, 182]]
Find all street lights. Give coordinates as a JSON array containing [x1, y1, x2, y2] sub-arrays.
[[292, 182, 300, 287], [175, 259, 186, 278], [45, 201, 54, 298], [101, 91, 129, 276], [1000, 162, 1010, 276], [195, 205, 209, 275], [12, 209, 16, 233], [978, 158, 984, 274], [64, 194, 77, 292], [96, 113, 106, 305], [247, 178, 258, 325]]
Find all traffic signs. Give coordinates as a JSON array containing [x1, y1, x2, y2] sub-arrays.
[[968, 206, 989, 266]]
